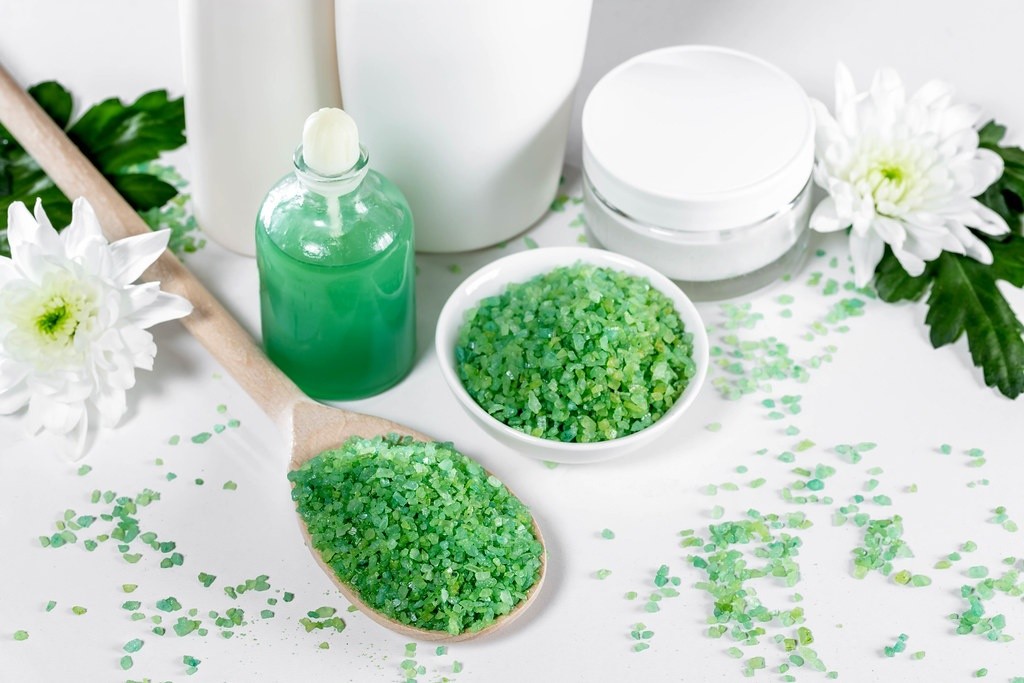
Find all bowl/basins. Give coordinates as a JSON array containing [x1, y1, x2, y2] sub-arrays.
[[581, 44, 814, 282], [437, 245, 709, 464]]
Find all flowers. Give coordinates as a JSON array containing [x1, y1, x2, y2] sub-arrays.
[[805, 56, 1010, 278], [0, 194, 196, 444]]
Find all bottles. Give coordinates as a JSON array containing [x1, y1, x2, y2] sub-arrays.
[[256, 108, 418, 403]]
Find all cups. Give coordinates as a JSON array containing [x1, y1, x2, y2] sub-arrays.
[[334, 0, 593, 253], [176, 0, 342, 258]]
